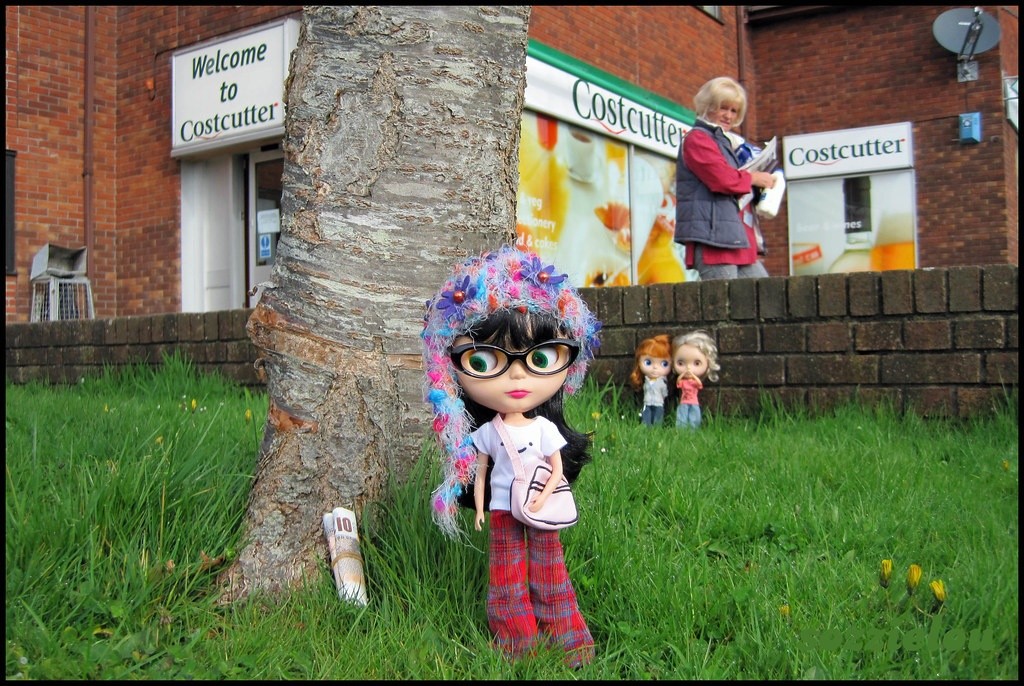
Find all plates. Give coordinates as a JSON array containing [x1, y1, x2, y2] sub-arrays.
[[564, 153, 603, 183]]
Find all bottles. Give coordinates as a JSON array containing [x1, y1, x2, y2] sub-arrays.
[[756, 166, 786, 219], [792, 242, 825, 276], [829, 176, 874, 274]]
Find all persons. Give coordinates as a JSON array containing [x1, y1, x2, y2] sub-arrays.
[[421, 247, 603, 671], [631, 334, 672, 430], [672, 331, 720, 429], [674, 76, 778, 282]]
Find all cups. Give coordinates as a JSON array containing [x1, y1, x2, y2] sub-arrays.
[[567, 130, 595, 177], [869, 214, 915, 272]]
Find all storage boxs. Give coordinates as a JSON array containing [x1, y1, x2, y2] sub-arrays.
[[31, 242, 87, 281]]
